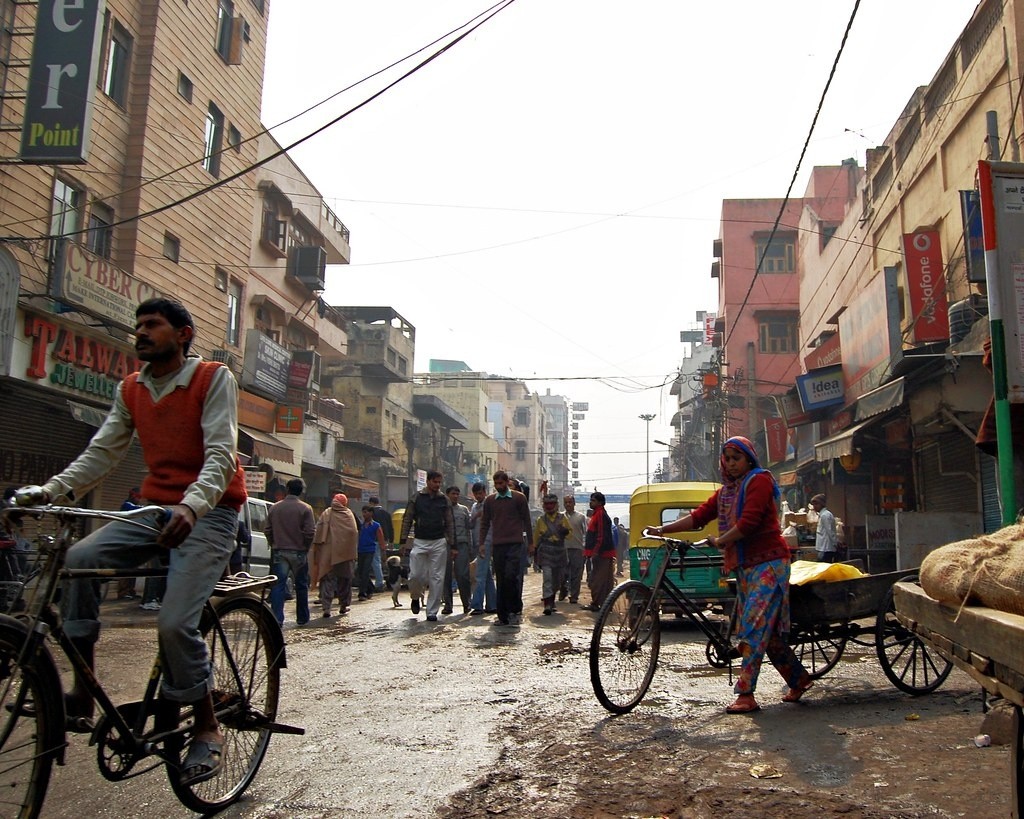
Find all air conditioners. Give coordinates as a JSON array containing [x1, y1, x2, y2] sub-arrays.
[[212, 349, 237, 371]]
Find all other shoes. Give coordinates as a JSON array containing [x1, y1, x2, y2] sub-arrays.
[[323, 612, 331, 618], [118, 592, 141, 600], [484, 609, 498, 613], [441, 607, 453, 614], [559, 591, 568, 600], [569, 597, 578, 603], [470, 609, 484, 615], [359, 595, 366, 601], [582, 604, 600, 611], [493, 620, 512, 627], [543, 604, 552, 616], [410, 599, 420, 614], [464, 603, 471, 612], [339, 608, 351, 614], [427, 615, 438, 622], [368, 586, 375, 598]]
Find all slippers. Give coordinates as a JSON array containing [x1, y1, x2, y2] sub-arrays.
[[6, 695, 93, 732], [784, 683, 816, 701], [726, 700, 762, 714], [185, 736, 231, 787]]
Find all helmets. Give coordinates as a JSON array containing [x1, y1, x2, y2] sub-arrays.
[[542, 494, 558, 514]]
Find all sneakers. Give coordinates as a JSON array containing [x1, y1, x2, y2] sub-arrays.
[[139, 599, 162, 609]]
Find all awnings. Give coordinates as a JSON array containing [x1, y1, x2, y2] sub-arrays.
[[11, 384, 139, 439], [853, 357, 943, 422], [814, 414, 883, 461], [238, 425, 293, 464]]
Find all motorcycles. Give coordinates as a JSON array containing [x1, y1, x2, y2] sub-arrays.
[[626, 481, 742, 627]]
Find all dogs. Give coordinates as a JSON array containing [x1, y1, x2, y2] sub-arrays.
[[386, 555, 428, 607]]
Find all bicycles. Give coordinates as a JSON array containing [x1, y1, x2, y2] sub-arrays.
[[1, 482, 313, 818]]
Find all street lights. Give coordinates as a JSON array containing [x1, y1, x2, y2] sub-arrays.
[[637, 413, 658, 503]]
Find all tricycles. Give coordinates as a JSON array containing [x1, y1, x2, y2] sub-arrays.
[[587, 525, 962, 716]]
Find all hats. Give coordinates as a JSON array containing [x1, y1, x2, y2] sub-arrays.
[[811, 493, 827, 507]]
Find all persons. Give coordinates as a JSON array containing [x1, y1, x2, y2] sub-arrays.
[[786, 428, 797, 454], [534, 493, 627, 615], [399, 469, 535, 625], [811, 494, 839, 563], [262, 478, 315, 629], [0, 296, 247, 785], [641, 436, 814, 713], [309, 490, 394, 618]]
